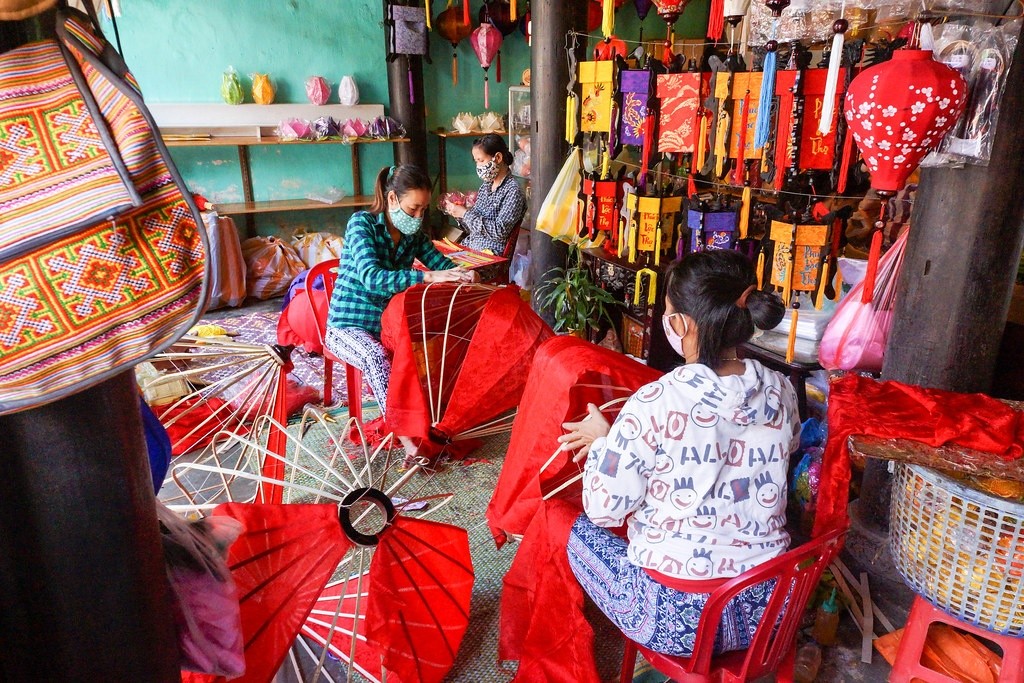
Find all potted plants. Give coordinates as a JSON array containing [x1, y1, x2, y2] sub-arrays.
[[533, 233, 628, 341]]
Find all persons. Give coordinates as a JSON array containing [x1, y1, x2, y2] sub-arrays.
[[324, 163, 481, 473], [0, 0, 185, 683], [557, 251, 802, 659], [445, 133, 528, 282]]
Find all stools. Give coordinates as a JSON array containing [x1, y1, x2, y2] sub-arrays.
[[888, 593, 1024, 683]]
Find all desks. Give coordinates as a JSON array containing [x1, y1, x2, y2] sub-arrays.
[[429, 126, 531, 224]]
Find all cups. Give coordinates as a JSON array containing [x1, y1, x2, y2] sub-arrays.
[[795, 639, 821, 683]]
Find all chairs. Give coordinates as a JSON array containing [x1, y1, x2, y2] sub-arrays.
[[619, 518, 852, 683], [485, 197, 528, 284], [305, 259, 375, 426]]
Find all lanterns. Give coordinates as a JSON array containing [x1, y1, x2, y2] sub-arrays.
[[383, 0, 432, 104], [470, 23, 503, 109], [843, 46, 967, 304], [562, 38, 918, 361], [434, 5, 473, 85], [520, 0, 750, 61], [479, 0, 519, 83]]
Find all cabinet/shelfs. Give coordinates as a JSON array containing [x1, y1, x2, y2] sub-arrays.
[[508, 86, 531, 179], [157, 137, 413, 236]]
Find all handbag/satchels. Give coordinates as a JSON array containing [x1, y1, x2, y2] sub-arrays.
[[819, 226, 910, 371], [535, 144, 597, 250]]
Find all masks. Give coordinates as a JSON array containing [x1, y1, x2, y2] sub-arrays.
[[476, 156, 499, 182], [662, 312, 688, 357], [388, 194, 423, 235]]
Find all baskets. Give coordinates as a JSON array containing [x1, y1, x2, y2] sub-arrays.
[[888, 461, 1024, 639]]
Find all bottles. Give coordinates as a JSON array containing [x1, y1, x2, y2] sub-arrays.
[[814, 587, 839, 645]]
